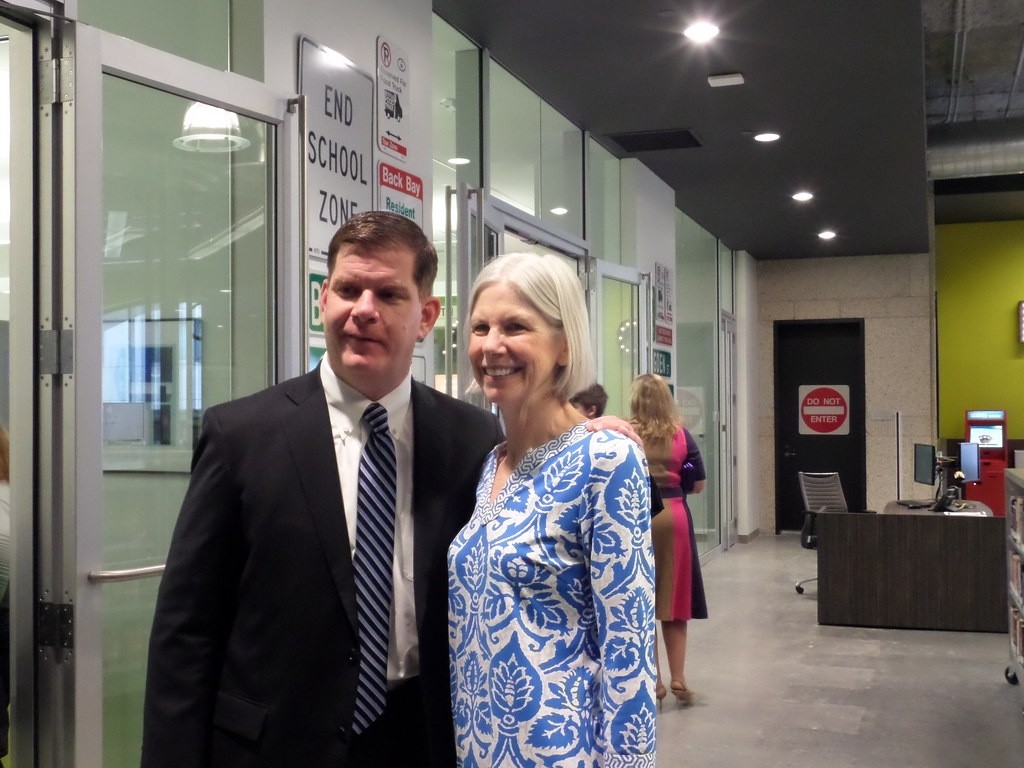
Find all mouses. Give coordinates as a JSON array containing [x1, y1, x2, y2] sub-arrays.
[[907, 503, 922, 509]]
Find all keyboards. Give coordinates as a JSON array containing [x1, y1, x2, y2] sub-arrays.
[[897, 500, 931, 506]]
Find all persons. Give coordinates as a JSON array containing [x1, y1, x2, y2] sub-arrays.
[[624, 372, 708, 713], [0, 422, 10, 768], [142, 211, 656, 768]]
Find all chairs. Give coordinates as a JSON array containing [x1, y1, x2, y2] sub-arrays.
[[794, 471, 876, 594]]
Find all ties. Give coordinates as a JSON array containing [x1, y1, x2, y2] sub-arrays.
[[352, 401, 397, 737]]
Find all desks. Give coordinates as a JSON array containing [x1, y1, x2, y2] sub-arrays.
[[815, 497, 1010, 634]]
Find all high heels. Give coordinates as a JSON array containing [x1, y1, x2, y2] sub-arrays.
[[670, 678, 692, 708], [655, 685, 666, 711]]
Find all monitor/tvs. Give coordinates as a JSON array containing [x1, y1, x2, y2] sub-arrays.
[[957, 442, 981, 483], [914, 443, 936, 485]]
